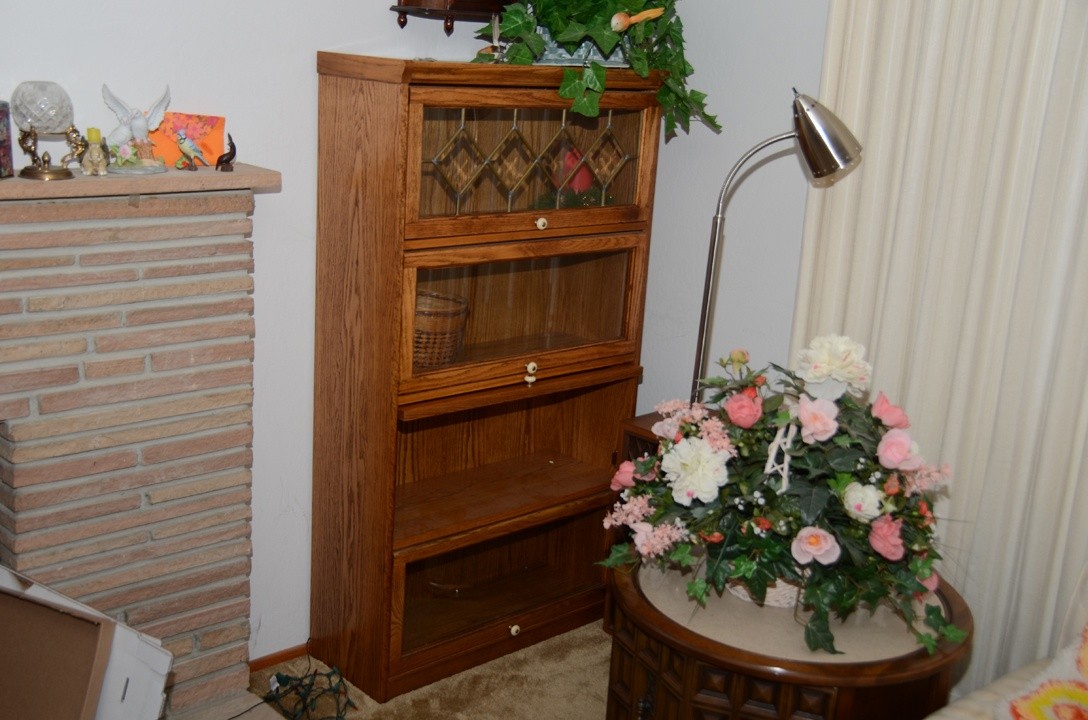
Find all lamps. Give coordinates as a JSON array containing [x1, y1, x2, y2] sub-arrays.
[[689, 87, 862, 407]]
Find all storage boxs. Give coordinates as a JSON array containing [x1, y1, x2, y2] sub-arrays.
[[0, 565, 174, 720]]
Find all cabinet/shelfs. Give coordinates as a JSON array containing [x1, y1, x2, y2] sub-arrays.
[[310, 52, 671, 703]]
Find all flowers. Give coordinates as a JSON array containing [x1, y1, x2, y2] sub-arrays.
[[111, 143, 137, 166], [593, 335, 969, 656]]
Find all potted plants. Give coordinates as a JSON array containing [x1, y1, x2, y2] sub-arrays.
[[471, 0, 723, 136]]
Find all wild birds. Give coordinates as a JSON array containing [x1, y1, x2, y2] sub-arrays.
[[176, 129, 210, 167], [102, 83, 171, 144]]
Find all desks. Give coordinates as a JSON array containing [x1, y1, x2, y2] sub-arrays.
[[604, 544, 977, 720], [622, 406, 722, 464]]
